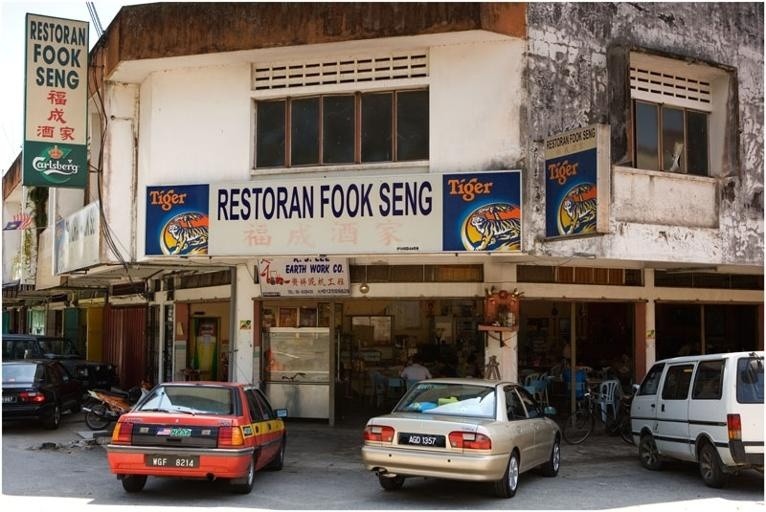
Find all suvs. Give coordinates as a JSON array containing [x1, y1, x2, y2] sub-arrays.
[[2, 333, 119, 413]]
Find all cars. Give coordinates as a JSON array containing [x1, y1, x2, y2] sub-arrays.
[[105, 377, 288, 495], [359, 377, 561, 499], [2, 359, 82, 431]]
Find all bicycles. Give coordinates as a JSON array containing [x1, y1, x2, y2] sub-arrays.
[[562, 388, 633, 445]]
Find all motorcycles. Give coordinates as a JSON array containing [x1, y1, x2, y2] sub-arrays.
[[81, 378, 154, 430]]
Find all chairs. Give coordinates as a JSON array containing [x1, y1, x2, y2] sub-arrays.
[[343, 349, 628, 424]]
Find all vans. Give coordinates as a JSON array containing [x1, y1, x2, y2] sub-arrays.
[[628, 350, 764, 488]]
